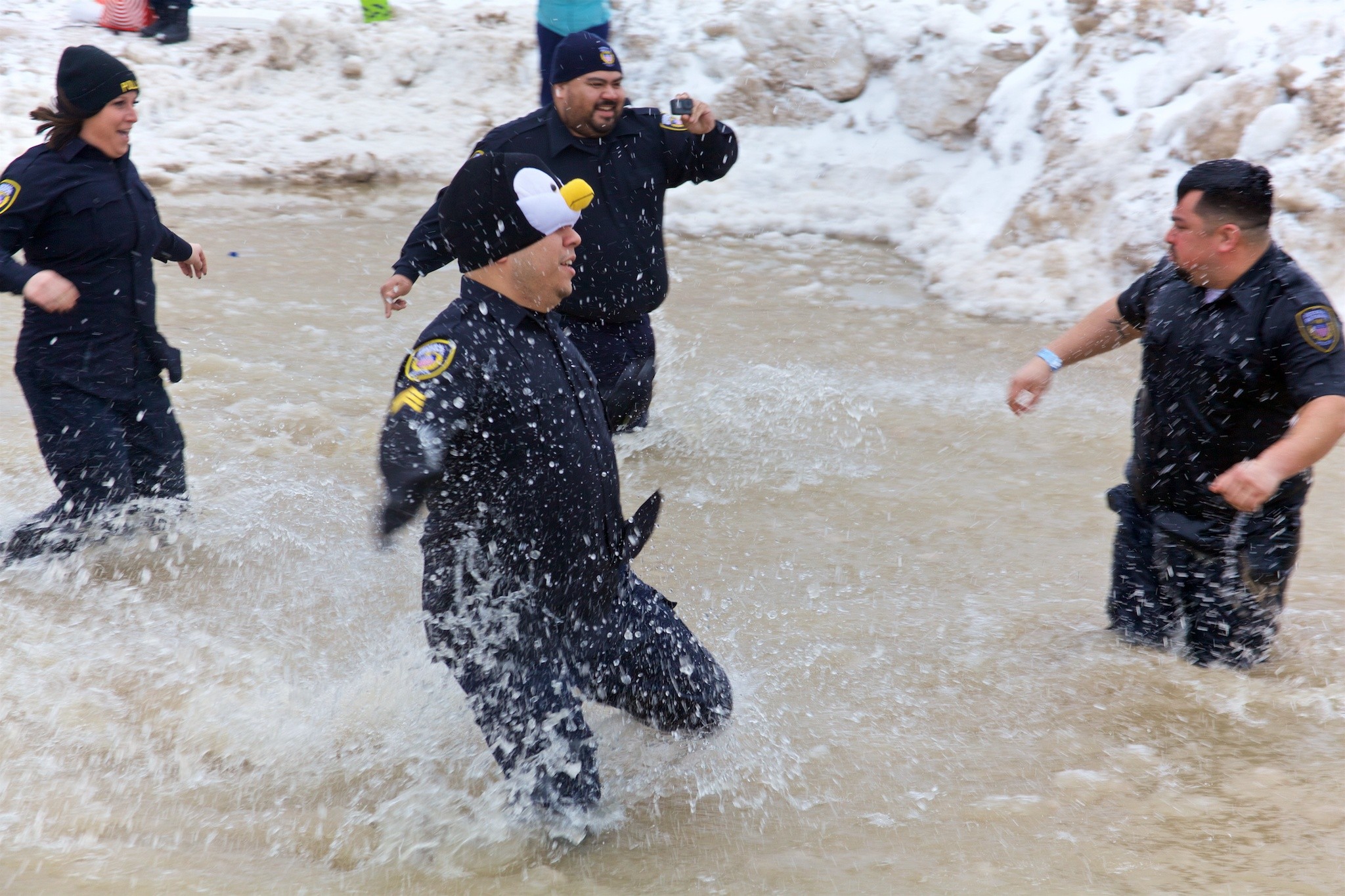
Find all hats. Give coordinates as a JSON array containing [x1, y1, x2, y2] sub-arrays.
[[550, 31, 623, 85], [437, 153, 595, 273], [56, 44, 140, 120]]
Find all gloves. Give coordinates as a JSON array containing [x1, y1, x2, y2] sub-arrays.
[[383, 467, 446, 540], [605, 357, 656, 429]]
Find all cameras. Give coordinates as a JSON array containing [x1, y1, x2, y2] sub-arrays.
[[670, 98, 693, 116]]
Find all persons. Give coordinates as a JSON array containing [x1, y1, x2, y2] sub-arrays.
[[364, 148, 733, 845], [380, 31, 739, 448], [532, 0, 626, 109], [1006, 157, 1344, 672], [137, 0, 198, 44], [0, 42, 208, 572]]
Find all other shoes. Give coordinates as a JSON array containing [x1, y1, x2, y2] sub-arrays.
[[141, 17, 189, 43]]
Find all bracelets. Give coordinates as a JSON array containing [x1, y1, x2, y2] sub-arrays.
[[1036, 348, 1063, 372]]
